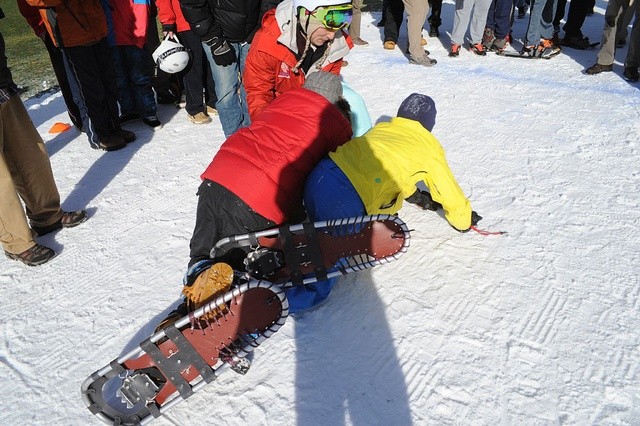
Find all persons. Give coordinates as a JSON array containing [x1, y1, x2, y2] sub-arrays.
[[243, 1, 353, 121], [186, 93, 483, 342], [402, 0, 437, 66], [519, 0, 561, 59], [482, 0, 513, 50], [585, 0, 640, 82], [183, 71, 352, 376], [156, 0, 218, 125], [384, 0, 429, 49], [16, 0, 137, 152], [377, 0, 442, 37], [347, 1, 369, 47], [102, 0, 164, 131], [512, 0, 535, 20], [560, 0, 596, 49], [0, 8, 87, 267], [179, 1, 284, 139], [448, 0, 493, 56]]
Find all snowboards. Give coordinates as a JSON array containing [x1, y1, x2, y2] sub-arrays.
[[80, 280, 290, 425], [210, 215, 410, 288]]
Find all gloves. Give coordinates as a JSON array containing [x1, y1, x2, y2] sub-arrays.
[[452, 211, 482, 232], [406, 186, 442, 210], [202, 33, 238, 66]]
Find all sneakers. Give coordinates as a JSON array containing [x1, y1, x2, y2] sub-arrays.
[[563, 31, 589, 47], [523, 46, 554, 57], [624, 67, 639, 81], [410, 58, 437, 66], [33, 209, 88, 236], [205, 105, 218, 116], [491, 38, 506, 51], [482, 27, 495, 51], [384, 40, 395, 49], [114, 127, 136, 142], [176, 93, 186, 108], [407, 46, 429, 56], [142, 116, 163, 130], [351, 37, 368, 44], [429, 26, 439, 36], [450, 43, 461, 56], [183, 262, 234, 313], [119, 113, 140, 125], [421, 37, 427, 45], [587, 63, 613, 73], [468, 42, 486, 55], [188, 112, 212, 123], [5, 243, 55, 266], [99, 134, 127, 150], [541, 38, 561, 54]]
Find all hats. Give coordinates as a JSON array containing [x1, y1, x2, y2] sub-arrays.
[[397, 92, 436, 131], [300, 70, 342, 105]]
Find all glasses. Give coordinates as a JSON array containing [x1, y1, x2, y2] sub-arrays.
[[300, 3, 353, 29]]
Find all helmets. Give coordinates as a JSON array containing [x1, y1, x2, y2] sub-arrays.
[[293, 0, 351, 14], [152, 39, 189, 73]]
